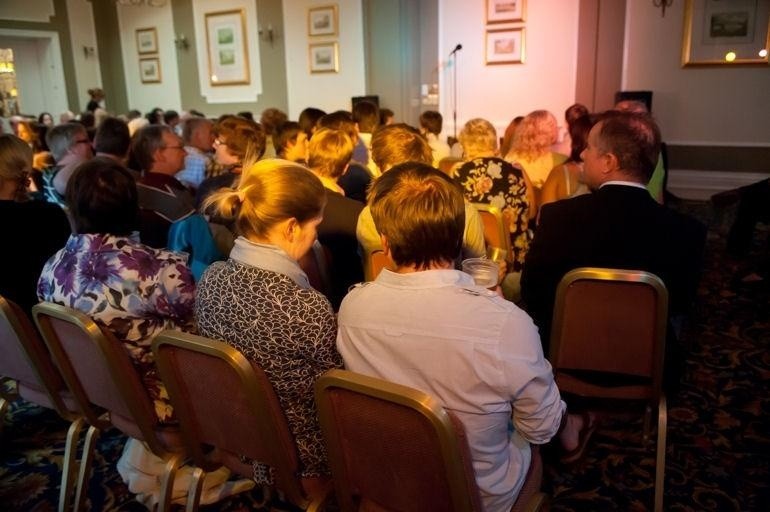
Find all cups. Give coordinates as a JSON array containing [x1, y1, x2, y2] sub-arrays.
[[461, 257, 499, 291]]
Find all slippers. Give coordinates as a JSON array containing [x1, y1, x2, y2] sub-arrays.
[[559, 409, 598, 466]]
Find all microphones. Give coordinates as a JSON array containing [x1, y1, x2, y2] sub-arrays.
[[450, 45, 462, 54]]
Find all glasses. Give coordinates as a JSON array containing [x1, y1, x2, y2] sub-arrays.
[[213, 137, 230, 147], [75, 138, 93, 144], [165, 144, 186, 152]]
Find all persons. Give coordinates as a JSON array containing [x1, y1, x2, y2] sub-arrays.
[[354, 125, 506, 300], [2, 133, 74, 306], [352, 101, 382, 139], [417, 110, 452, 166], [305, 128, 365, 253], [615, 97, 665, 206], [319, 111, 372, 199], [63, 85, 183, 140], [298, 108, 326, 133], [272, 122, 310, 163], [378, 108, 397, 126], [38, 158, 194, 455], [544, 103, 593, 158], [128, 126, 194, 246], [175, 117, 217, 187], [454, 115, 535, 267], [195, 117, 267, 209], [92, 119, 131, 165], [43, 123, 95, 206], [522, 111, 709, 394], [541, 115, 602, 202], [17, 112, 53, 149], [507, 111, 573, 190], [221, 109, 287, 131], [334, 165, 598, 510], [195, 155, 344, 487]]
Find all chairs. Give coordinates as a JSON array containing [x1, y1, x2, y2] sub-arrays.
[[151, 330, 313, 512], [476, 204, 506, 264], [548, 266, 668, 505], [317, 369, 484, 512], [0, 296, 86, 512], [30, 302, 158, 512]]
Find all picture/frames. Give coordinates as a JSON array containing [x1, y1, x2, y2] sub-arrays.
[[681, 0, 770, 68], [205, 8, 250, 86], [308, 4, 338, 37], [308, 42, 339, 73], [484, 0, 525, 25], [140, 58, 161, 84], [135, 27, 158, 54], [485, 29, 526, 63]]
